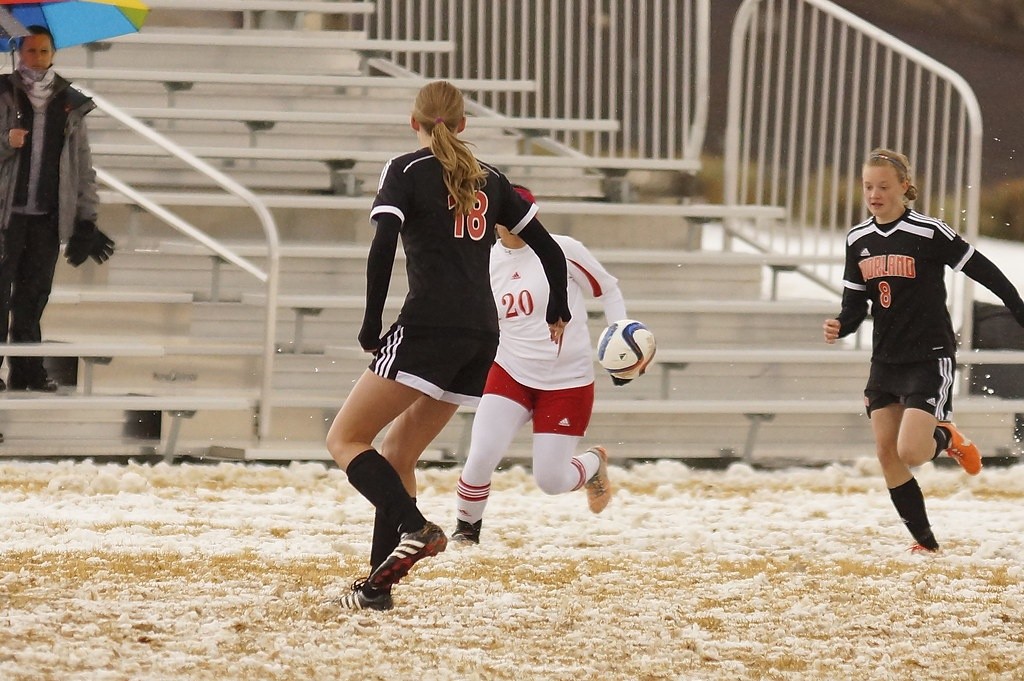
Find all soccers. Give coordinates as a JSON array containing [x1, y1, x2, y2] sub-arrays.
[[597, 319, 658, 378]]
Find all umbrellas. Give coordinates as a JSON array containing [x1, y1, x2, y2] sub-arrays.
[[0, 0, 150, 129]]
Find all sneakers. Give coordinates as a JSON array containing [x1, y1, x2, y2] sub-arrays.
[[904, 544, 936, 555], [584, 446, 612, 514], [935, 420, 983, 476], [447, 539, 480, 551], [333, 576, 394, 611], [368, 520, 448, 590]]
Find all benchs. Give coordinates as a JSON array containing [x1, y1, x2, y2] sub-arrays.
[[0, 0, 1024, 462]]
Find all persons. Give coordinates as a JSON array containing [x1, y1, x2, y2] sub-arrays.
[[327, 82, 573, 610], [450, 185, 630, 548], [823, 148, 1024, 559], [0, 25, 99, 395]]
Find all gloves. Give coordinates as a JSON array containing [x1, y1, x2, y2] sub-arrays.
[[64, 220, 98, 268], [72, 225, 115, 265]]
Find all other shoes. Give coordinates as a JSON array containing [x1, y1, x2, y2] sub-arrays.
[[6, 372, 58, 392], [0, 433, 4, 443], [0, 378, 6, 391]]
